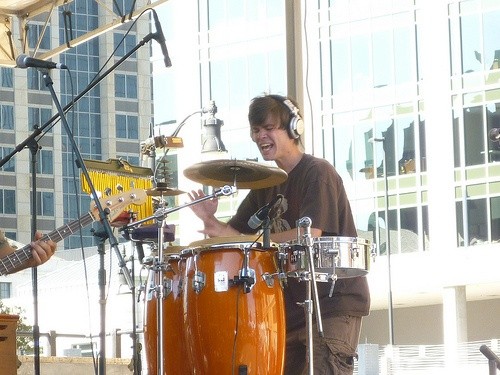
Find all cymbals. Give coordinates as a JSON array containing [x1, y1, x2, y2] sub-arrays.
[[183, 159, 288, 189]]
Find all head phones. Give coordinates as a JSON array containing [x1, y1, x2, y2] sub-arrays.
[[250, 95, 305, 143]]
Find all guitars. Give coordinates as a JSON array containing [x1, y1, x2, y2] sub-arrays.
[[0, 188, 148, 277]]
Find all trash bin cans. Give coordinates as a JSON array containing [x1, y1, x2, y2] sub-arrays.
[[0, 314, 20, 375]]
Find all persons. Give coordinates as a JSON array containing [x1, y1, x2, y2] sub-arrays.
[[0, 230, 57, 275], [187, 95, 371, 375]]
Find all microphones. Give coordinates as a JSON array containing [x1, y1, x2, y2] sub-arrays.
[[16, 54, 67, 69], [248, 194, 282, 230], [152, 9, 173, 68]]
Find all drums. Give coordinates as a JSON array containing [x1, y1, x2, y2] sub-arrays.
[[283, 236, 371, 279], [143, 246, 190, 375], [179, 242, 286, 375]]
